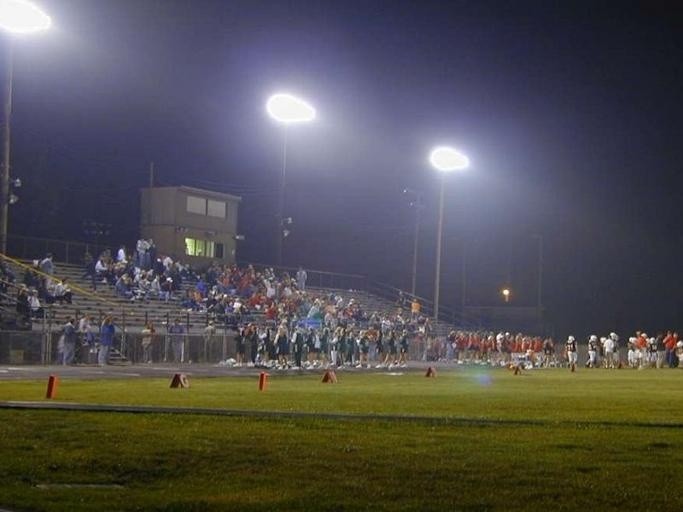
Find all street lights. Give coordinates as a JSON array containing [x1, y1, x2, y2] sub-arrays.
[[266, 92, 316, 269], [0, 2, 50, 260], [429, 146, 463, 320]]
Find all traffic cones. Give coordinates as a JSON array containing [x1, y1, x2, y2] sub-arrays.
[[259, 372, 264, 392], [46, 375, 56, 398]]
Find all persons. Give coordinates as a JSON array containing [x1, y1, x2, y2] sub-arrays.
[[1, 238, 437, 371], [422, 330, 682, 371]]
